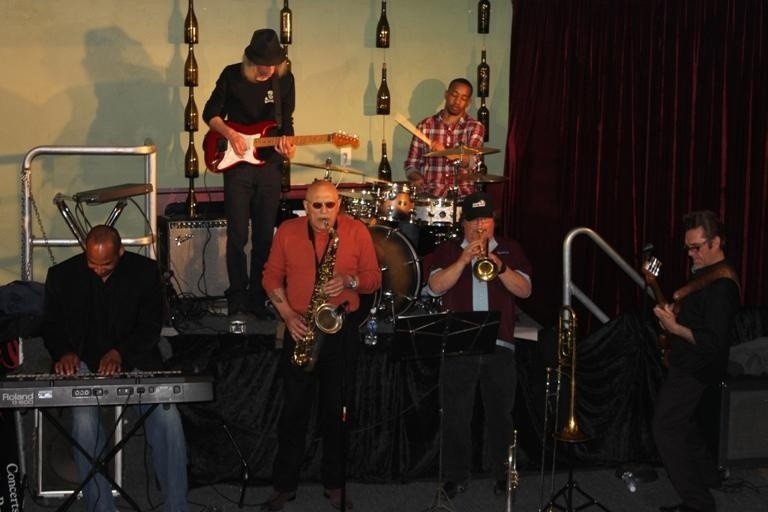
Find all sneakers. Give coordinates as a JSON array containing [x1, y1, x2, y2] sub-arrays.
[[259, 489, 297, 512], [322, 487, 355, 512]]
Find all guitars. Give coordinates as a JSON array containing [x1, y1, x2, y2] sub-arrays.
[[203, 122, 360, 172], [642, 256, 673, 365]]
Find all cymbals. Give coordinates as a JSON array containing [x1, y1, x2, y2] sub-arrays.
[[424, 147, 498, 157], [459, 174, 509, 183], [289, 160, 364, 177]]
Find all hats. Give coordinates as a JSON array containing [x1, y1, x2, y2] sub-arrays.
[[460, 190, 496, 220], [242, 26, 289, 66]]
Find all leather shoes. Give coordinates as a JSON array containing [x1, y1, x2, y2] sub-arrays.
[[227, 303, 251, 319], [247, 303, 277, 322], [657, 503, 698, 512], [492, 477, 509, 499], [437, 479, 471, 502]]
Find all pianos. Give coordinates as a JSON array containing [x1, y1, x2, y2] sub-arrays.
[[0, 371, 215, 408]]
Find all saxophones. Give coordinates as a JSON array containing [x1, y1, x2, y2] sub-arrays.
[[294, 221, 345, 368]]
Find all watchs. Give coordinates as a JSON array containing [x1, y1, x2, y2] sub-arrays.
[[348, 275, 358, 291], [497, 261, 509, 276]]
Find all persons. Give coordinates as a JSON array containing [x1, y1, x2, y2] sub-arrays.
[[404, 80, 487, 198], [203, 28, 295, 321], [651, 210, 741, 512], [421, 191, 536, 501], [44, 225, 189, 512], [261, 181, 382, 512]]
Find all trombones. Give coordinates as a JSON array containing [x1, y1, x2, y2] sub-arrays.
[[538, 304, 592, 511], [505, 428, 522, 512]]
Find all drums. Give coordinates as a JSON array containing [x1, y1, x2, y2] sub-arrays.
[[364, 223, 425, 322], [341, 191, 373, 218], [376, 185, 416, 223], [411, 199, 464, 229]]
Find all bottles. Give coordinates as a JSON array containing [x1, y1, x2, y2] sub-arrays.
[[279, 192, 289, 223], [376, 68, 391, 115], [283, 45, 291, 73], [281, 154, 290, 192], [279, 0, 292, 44], [365, 317, 378, 345], [479, 155, 486, 192], [184, 0, 198, 218], [378, 140, 391, 185], [476, 0, 490, 143], [375, 0, 390, 49]]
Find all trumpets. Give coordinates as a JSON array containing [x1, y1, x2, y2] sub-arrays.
[[471, 230, 497, 283]]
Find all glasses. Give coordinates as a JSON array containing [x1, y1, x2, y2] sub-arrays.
[[680, 239, 713, 254], [303, 196, 342, 211]]
